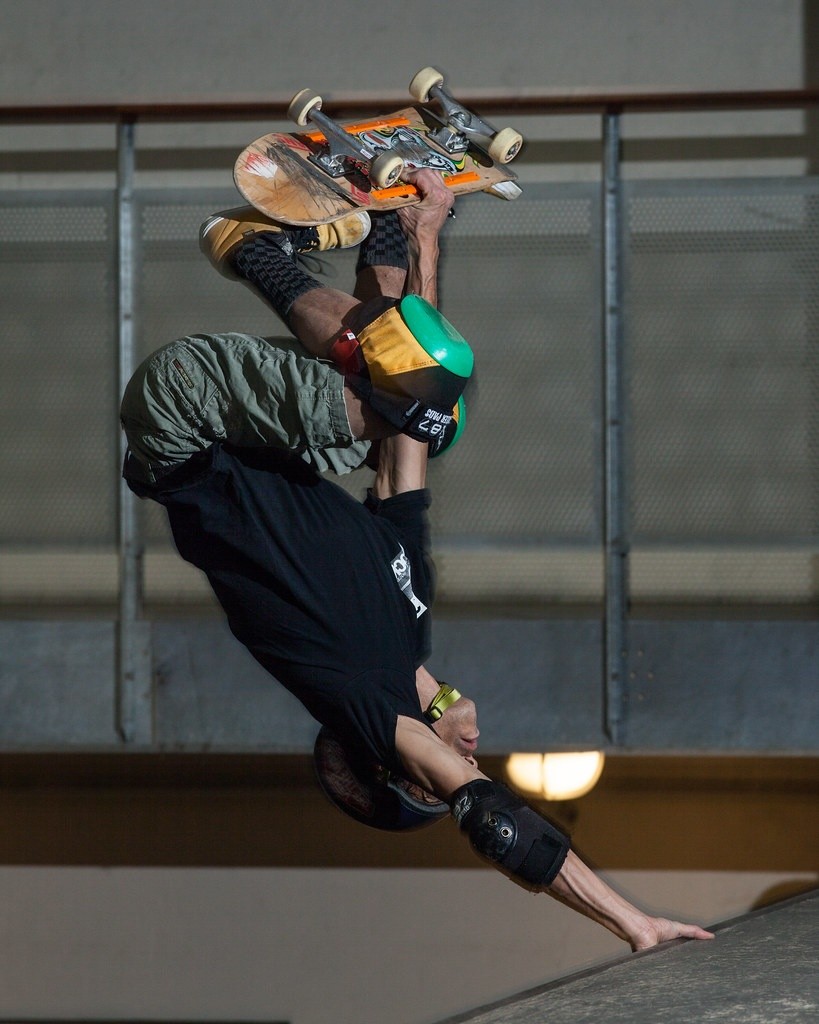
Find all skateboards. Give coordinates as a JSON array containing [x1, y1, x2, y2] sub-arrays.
[[233, 64, 527, 225]]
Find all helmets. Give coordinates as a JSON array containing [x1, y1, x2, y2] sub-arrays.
[[313, 725, 451, 832]]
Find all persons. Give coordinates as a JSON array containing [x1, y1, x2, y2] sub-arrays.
[[120, 164, 715, 955]]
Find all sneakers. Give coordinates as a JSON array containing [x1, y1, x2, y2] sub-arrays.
[[447, 181, 523, 218], [199, 205, 371, 282]]
